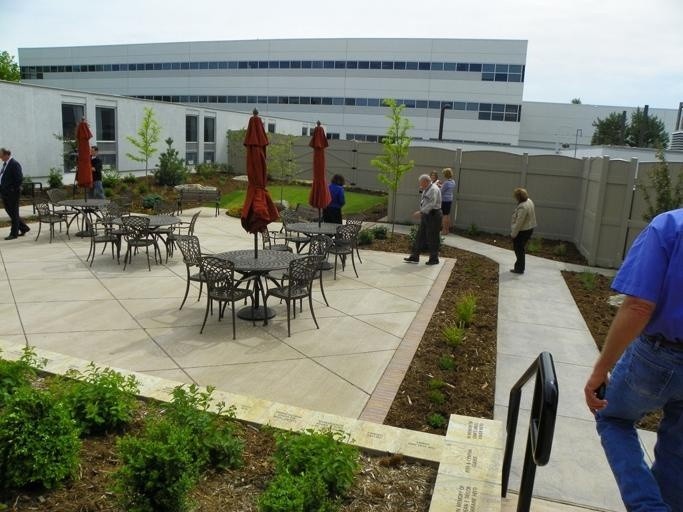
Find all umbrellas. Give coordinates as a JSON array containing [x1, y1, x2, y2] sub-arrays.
[[308, 118, 334, 271], [76, 115, 100, 239], [237, 106, 278, 321]]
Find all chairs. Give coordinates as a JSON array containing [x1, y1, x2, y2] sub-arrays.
[[169, 203, 365, 344], [33, 187, 203, 272]]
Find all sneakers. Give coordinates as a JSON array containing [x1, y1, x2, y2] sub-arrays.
[[404, 257, 419, 262], [426, 260, 439, 264]]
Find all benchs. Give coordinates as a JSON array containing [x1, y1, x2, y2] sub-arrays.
[[174, 189, 223, 217]]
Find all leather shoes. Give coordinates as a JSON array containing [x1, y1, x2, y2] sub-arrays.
[[19, 227, 30, 236], [5, 235, 17, 239]]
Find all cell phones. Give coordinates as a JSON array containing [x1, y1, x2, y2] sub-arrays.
[[596, 382, 606, 399]]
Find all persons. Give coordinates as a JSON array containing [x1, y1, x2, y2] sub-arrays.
[[88, 145, 107, 200], [440, 167, 456, 236], [0, 147, 32, 242], [583, 206, 683, 511], [322, 173, 346, 223], [429, 169, 443, 188], [402, 174, 443, 265], [509, 188, 537, 274]]
